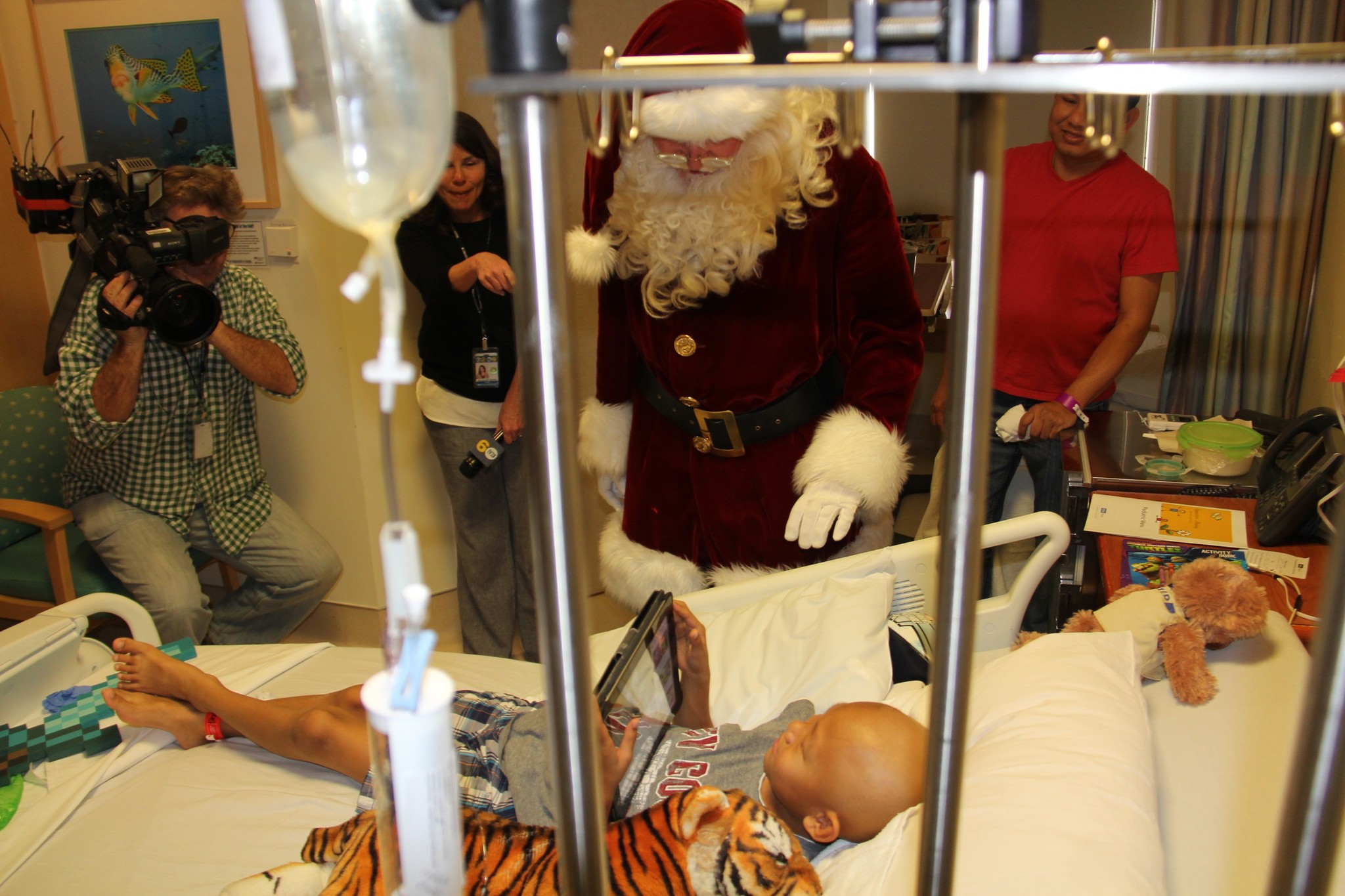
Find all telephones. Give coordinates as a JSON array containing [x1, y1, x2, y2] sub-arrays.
[[1253, 406, 1345, 547]]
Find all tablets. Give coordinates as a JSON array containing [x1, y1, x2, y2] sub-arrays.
[[592, 591, 686, 818]]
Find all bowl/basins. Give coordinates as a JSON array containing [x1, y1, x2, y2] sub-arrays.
[[1176, 422, 1263, 477]]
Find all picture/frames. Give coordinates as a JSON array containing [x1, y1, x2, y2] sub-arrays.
[[26, 0, 283, 212]]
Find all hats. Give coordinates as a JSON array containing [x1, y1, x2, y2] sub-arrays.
[[563, 1, 771, 278]]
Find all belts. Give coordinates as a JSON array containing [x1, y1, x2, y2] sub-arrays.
[[630, 364, 841, 460]]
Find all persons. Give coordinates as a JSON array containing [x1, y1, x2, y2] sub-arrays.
[[562, 1, 928, 619], [398, 107, 541, 666], [476, 365, 488, 379], [917, 42, 1182, 636], [104, 597, 925, 856], [53, 165, 349, 653]]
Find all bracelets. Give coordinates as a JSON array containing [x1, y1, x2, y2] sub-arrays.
[[1055, 391, 1091, 424]]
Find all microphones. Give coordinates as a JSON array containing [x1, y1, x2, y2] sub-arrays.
[[117, 234, 158, 278], [459, 428, 507, 477]]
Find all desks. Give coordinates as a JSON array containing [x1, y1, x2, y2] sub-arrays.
[[1054, 409, 1338, 642], [1090, 490, 1330, 644]]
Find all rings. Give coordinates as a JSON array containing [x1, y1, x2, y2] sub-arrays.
[[517, 432, 524, 437]]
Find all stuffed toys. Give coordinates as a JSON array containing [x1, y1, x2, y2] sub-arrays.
[[1010, 557, 1270, 706], [222, 784, 825, 896]]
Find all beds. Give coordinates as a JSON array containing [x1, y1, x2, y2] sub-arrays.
[[1, 506, 1345, 896]]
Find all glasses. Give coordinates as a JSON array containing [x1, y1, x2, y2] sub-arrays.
[[653, 139, 742, 173], [160, 216, 240, 242]]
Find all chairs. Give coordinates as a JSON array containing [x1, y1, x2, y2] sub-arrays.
[[0, 383, 240, 639]]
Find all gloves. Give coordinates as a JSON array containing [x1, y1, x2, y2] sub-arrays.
[[597, 470, 625, 512], [782, 481, 864, 550]]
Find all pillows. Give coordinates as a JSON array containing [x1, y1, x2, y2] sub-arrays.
[[587, 573, 896, 730], [878, 629, 1167, 896]]
